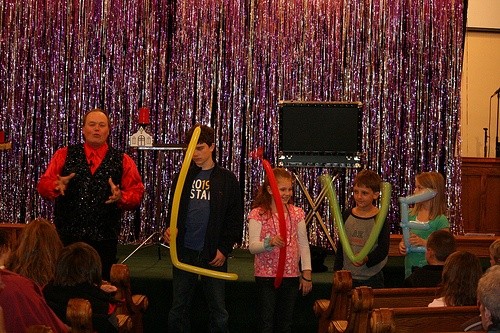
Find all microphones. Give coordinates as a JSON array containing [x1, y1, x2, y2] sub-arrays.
[[491, 88, 500, 98]]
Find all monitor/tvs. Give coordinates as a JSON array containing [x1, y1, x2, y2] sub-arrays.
[[277, 101, 363, 168]]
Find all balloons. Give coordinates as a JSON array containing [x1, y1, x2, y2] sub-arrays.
[[249, 146, 287, 289], [398, 187, 437, 255], [169, 125, 239, 281], [320, 173, 392, 265]]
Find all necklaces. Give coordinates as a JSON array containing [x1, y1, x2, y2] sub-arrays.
[[271, 204, 293, 247]]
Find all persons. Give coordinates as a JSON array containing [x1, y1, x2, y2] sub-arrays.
[[399, 170, 449, 275], [46, 241, 121, 333], [248, 168, 313, 332], [476, 265, 500, 333], [3, 216, 63, 285], [396, 228, 458, 287], [330, 171, 391, 298], [428, 251, 484, 307], [489, 239, 500, 267], [165, 122, 244, 333], [36, 108, 144, 282], [0, 229, 69, 333]]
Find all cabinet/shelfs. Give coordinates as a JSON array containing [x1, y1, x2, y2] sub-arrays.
[[462, 157, 500, 234]]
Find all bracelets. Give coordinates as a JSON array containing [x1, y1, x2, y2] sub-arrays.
[[301, 275, 312, 283]]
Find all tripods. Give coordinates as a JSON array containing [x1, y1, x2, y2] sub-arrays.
[[121, 150, 170, 264]]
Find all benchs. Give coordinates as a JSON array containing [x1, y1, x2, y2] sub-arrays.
[[62, 298, 97, 332], [366, 306, 481, 333], [327, 286, 480, 333], [98, 285, 133, 333], [313, 270, 442, 333], [110, 264, 149, 333]]
